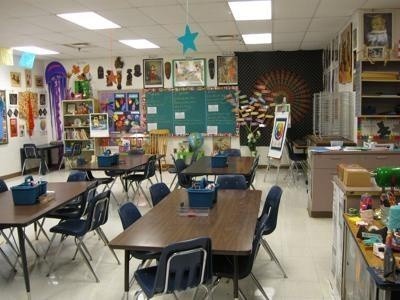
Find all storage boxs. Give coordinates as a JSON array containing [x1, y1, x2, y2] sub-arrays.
[[336, 164, 363, 181], [344, 169, 374, 187]]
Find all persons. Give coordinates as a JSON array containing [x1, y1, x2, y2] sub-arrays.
[[149, 64, 157, 80], [229, 57, 238, 83], [365, 14, 389, 47], [218, 61, 228, 83]]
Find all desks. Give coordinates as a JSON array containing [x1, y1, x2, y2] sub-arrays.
[[1, 182, 98, 293], [342, 214, 400, 300], [107, 189, 263, 298], [305, 134, 357, 170], [329, 175, 399, 300]]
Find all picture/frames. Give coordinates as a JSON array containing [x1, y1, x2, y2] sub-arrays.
[[217, 56, 239, 86], [172, 58, 208, 88], [142, 58, 165, 89]]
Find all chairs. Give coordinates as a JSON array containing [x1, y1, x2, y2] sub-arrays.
[[46, 189, 120, 284], [42, 188, 96, 261], [204, 215, 268, 298], [36, 173, 94, 240], [148, 183, 170, 206], [253, 186, 290, 278], [131, 237, 213, 298], [147, 129, 170, 172], [117, 204, 158, 289], [118, 155, 159, 208], [1, 180, 50, 256], [15, 138, 309, 191]]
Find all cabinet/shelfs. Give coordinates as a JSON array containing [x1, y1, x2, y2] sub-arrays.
[[361, 152, 400, 171], [356, 58, 399, 118], [61, 99, 98, 168], [308, 147, 359, 218]]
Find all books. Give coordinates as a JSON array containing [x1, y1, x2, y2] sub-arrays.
[[65, 128, 90, 152]]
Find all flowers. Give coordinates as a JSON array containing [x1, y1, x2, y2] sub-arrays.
[[248, 129, 262, 151]]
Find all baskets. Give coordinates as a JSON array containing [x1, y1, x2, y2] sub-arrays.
[[211, 150, 228, 168], [98, 150, 119, 166], [188, 178, 218, 207], [12, 176, 48, 204]]
